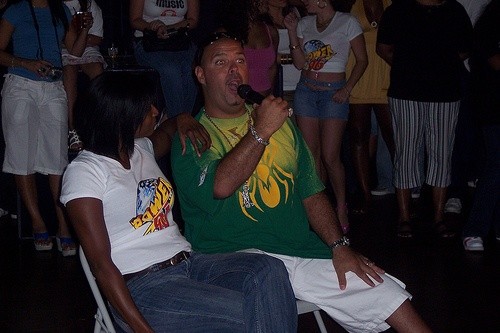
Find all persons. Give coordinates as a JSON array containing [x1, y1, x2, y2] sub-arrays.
[[168, 31, 433, 333], [0, 0, 500, 258], [59, 73, 298, 333]]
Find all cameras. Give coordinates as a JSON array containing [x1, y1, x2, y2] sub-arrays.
[[47, 68, 63, 80], [163, 28, 178, 37]]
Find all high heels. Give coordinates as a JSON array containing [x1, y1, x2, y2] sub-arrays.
[[32, 229, 53, 250], [56, 235, 76, 256]]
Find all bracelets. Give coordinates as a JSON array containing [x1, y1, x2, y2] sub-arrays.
[[289, 45, 300, 50], [342, 85, 351, 97], [249, 124, 269, 146]]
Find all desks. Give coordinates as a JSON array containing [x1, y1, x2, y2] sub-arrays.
[[77, 54, 161, 151]]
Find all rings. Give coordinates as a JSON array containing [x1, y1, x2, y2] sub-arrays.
[[366, 261, 369, 266], [287, 107, 293, 118]]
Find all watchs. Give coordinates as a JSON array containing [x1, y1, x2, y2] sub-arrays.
[[330, 236, 349, 250]]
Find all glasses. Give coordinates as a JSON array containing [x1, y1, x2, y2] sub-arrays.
[[197, 32, 243, 66]]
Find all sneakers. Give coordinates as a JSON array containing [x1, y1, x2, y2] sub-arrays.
[[444, 197, 462, 213], [463, 235, 484, 250]]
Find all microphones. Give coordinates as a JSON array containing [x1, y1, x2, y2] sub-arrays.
[[237, 84, 295, 118]]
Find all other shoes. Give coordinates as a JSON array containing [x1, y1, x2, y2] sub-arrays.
[[336, 205, 349, 235], [410, 187, 421, 198], [371, 187, 394, 196], [467, 177, 478, 187]]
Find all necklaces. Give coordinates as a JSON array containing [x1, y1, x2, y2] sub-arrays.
[[316, 11, 336, 30], [202, 106, 252, 150], [125, 146, 143, 184]]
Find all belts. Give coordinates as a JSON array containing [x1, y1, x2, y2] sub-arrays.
[[123, 252, 189, 281]]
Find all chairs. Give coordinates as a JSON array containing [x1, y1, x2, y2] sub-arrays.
[[77, 244, 328, 333]]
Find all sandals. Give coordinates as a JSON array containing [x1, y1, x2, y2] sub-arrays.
[[396, 221, 412, 241], [434, 221, 450, 241], [349, 195, 367, 219], [68, 129, 83, 150]]
